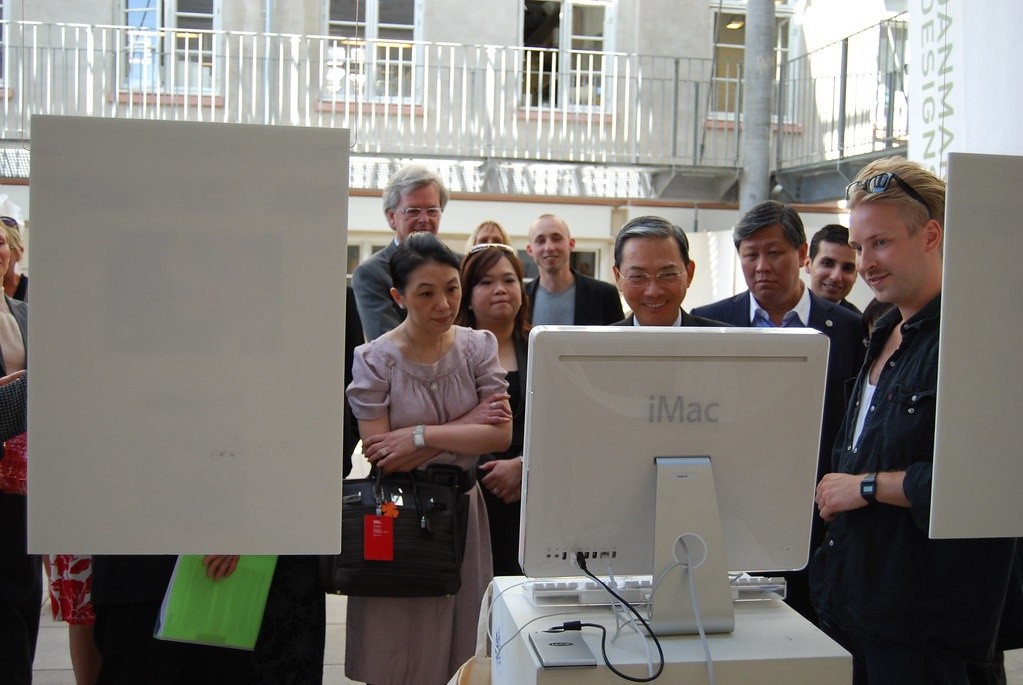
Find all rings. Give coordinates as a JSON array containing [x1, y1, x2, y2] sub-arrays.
[[495, 487, 502, 493], [379, 447, 387, 455], [490, 401, 498, 409]]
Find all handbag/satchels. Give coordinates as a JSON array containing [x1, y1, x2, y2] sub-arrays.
[[324, 459, 470, 599], [0, 370, 27, 442]]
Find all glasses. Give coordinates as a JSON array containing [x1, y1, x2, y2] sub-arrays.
[[615, 266, 689, 287], [845, 172, 933, 222], [466, 244, 516, 266], [396, 205, 442, 219]]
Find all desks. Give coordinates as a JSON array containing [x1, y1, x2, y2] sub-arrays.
[[476, 575, 853, 685]]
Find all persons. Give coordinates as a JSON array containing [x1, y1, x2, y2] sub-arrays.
[[0, 189, 359, 685], [351, 163, 463, 342], [524, 0, 554, 105], [862, 293, 898, 344], [465, 220, 514, 246], [337, 229, 515, 685], [803, 223, 863, 316], [815, 156, 1010, 672], [689, 201, 863, 474], [462, 246, 528, 587], [517, 214, 626, 402], [600, 217, 736, 327], [579, 262, 593, 277]]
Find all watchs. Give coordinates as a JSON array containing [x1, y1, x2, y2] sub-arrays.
[[413, 425, 426, 450], [859, 471, 883, 508]]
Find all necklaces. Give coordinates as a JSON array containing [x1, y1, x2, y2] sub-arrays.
[[403, 323, 447, 393]]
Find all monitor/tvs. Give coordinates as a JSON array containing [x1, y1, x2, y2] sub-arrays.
[[519, 325, 831, 637]]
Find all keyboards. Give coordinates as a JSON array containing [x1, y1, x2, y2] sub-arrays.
[[521, 577, 787, 606]]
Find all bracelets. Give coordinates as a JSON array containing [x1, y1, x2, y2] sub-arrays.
[[519, 456, 524, 463]]
